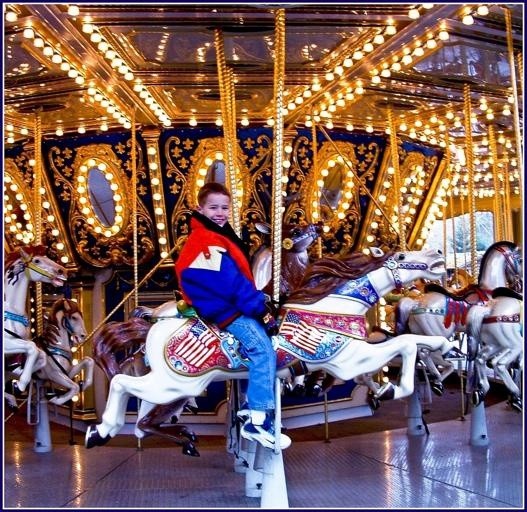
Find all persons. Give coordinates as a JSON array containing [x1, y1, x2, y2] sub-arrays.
[[174, 183, 292, 451]]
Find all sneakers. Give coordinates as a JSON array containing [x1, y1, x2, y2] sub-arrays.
[[236, 400, 254, 421], [242, 418, 291, 451]]
